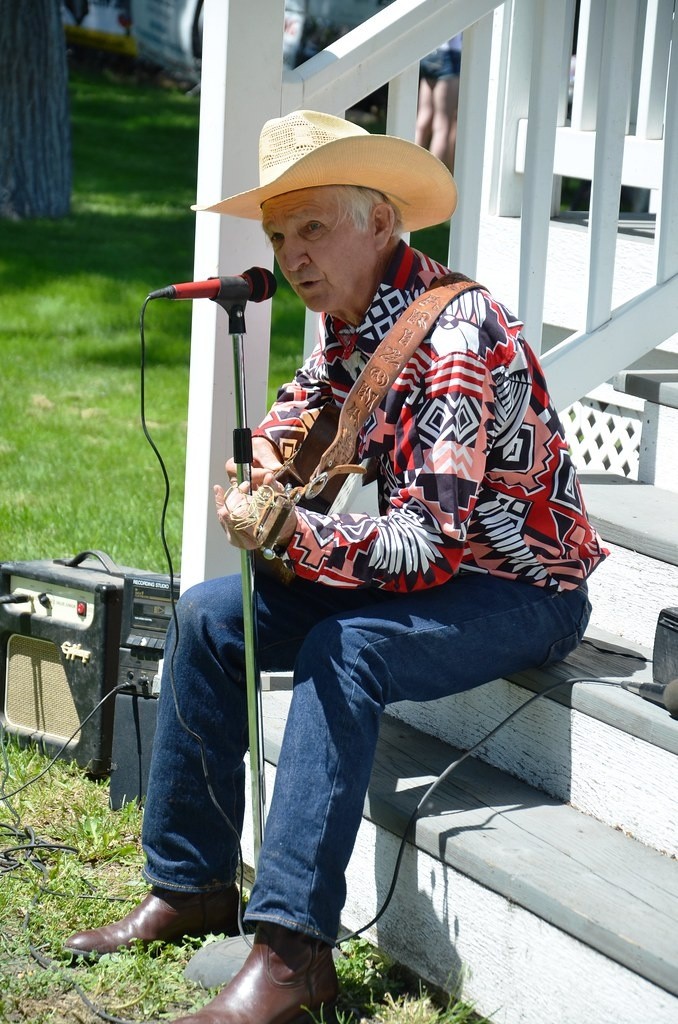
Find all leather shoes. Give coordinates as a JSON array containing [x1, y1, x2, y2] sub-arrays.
[[63, 880, 242, 958], [171, 920, 341, 1024]]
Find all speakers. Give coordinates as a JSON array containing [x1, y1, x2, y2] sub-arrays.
[[652, 608, 678, 685], [0, 557, 154, 777]]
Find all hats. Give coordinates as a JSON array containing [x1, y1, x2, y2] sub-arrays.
[[191, 109, 457, 236]]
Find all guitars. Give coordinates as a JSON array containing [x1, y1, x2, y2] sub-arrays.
[[222, 400, 363, 586]]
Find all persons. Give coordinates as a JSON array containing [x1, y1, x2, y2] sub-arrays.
[[65, 110, 609, 1024], [415, 32, 462, 172]]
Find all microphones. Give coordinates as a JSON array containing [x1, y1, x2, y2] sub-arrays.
[[148, 267, 277, 302], [620, 679, 678, 720]]
